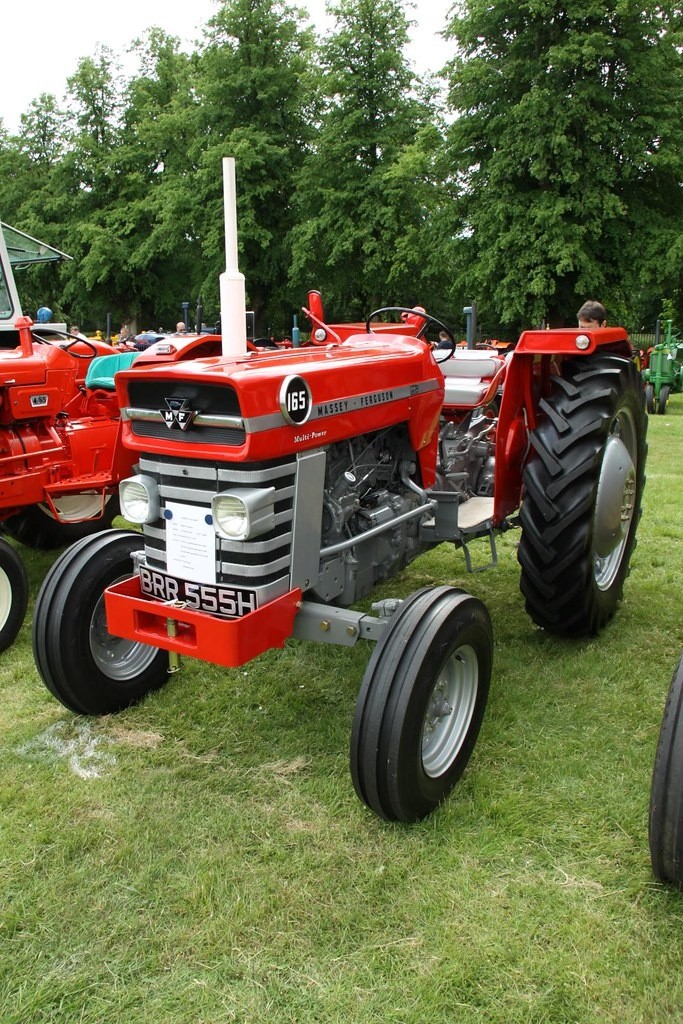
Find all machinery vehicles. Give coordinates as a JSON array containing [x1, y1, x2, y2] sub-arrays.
[[649, 658, 683, 887], [640, 318, 683, 415], [30, 157, 649, 824], [0, 219, 262, 654]]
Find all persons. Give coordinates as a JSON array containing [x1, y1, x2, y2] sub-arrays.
[[430, 331, 452, 349], [118, 327, 135, 348], [175, 321, 186, 335], [576, 300, 608, 328], [70, 326, 88, 340]]
